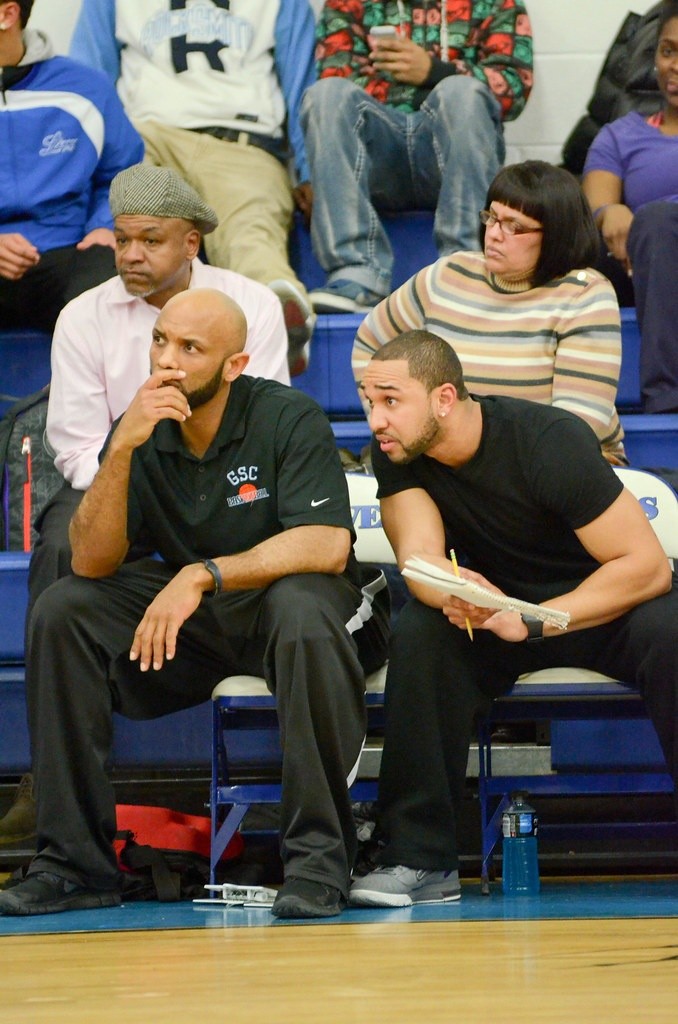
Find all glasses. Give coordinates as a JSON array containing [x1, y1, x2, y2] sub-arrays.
[[478, 209, 543, 235]]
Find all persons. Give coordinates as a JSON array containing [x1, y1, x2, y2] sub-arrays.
[[0, 0, 145, 332], [348, 329, 678, 907], [27, 0, 315, 377], [555, 0, 678, 416], [350, 159, 628, 467], [0, 160, 290, 846], [0, 286, 366, 919], [298, 0, 535, 316]]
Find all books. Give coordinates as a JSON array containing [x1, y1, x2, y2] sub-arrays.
[[398, 553, 572, 631]]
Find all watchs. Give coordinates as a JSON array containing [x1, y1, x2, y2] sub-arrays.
[[519, 613, 545, 643]]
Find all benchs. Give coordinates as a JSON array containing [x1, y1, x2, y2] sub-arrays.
[[0, 209, 678, 773]]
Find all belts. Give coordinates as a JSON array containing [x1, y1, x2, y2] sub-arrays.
[[195, 128, 291, 162]]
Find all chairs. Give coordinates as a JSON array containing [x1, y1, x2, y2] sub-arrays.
[[475, 465, 678, 892], [202, 476, 386, 895]]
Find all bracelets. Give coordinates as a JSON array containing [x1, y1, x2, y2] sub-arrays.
[[593, 204, 609, 218], [198, 556, 223, 602]]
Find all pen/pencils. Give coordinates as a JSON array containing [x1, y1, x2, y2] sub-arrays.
[[450, 549, 473, 641]]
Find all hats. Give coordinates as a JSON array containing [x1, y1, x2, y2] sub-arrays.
[[108, 162, 218, 235]]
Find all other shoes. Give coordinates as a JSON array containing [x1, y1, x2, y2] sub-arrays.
[[269, 278, 317, 378], [308, 278, 384, 314]]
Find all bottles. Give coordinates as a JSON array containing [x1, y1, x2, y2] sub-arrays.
[[502, 791, 540, 896]]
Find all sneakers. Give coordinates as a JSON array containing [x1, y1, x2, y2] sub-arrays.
[[270, 875, 348, 917], [348, 864, 462, 907], [0, 869, 122, 914]]
